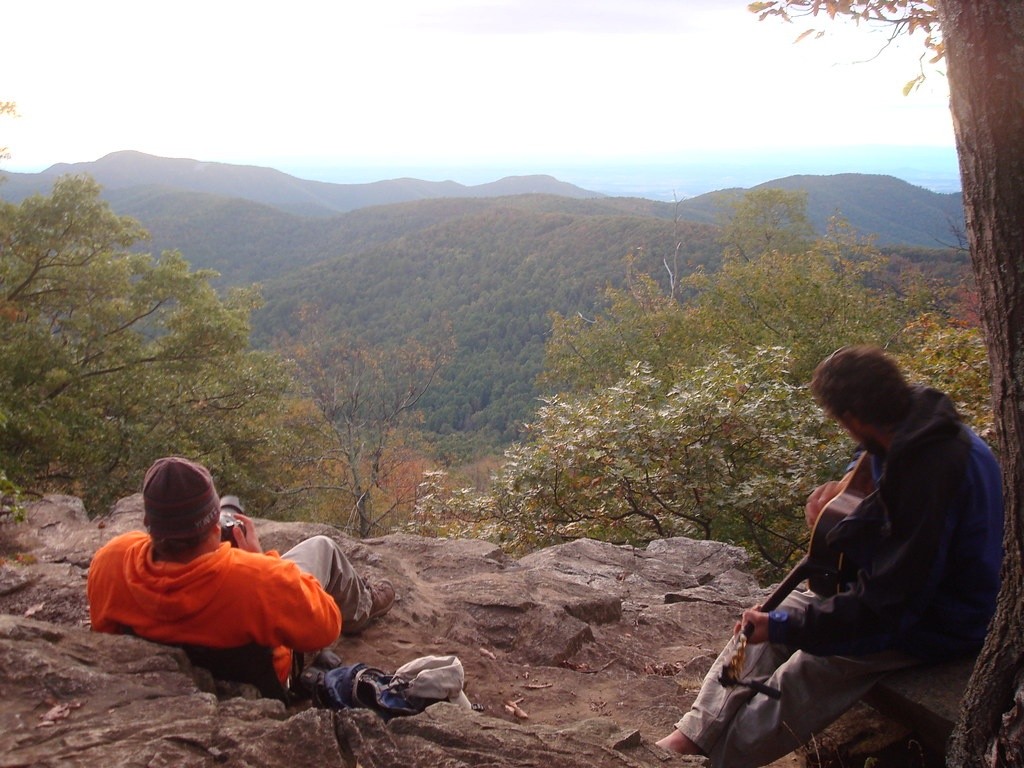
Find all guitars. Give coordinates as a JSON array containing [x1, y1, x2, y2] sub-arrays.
[[717, 448, 881, 700]]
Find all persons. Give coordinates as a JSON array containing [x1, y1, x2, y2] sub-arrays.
[[654, 347, 1001, 767], [88, 458, 395, 694]]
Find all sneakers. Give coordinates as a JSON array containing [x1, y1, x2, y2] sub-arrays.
[[338, 577, 395, 636]]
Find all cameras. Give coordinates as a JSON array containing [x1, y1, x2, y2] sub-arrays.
[[216, 495, 246, 549]]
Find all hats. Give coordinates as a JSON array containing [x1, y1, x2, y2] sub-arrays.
[[143, 458, 221, 539]]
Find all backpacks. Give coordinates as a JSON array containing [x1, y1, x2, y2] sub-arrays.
[[309, 662, 421, 718]]
